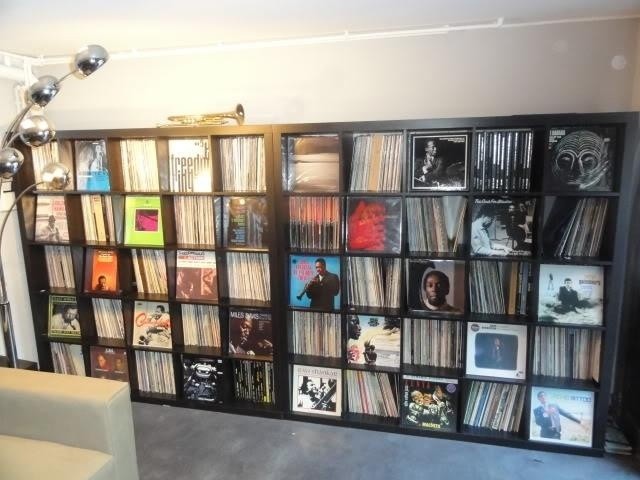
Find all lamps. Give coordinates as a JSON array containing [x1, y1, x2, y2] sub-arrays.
[[0, 164, 71, 255], [1, 46, 108, 224]]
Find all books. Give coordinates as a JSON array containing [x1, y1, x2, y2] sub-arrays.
[[293, 132, 470, 432], [75, 137, 175, 394], [528, 131, 619, 442], [169, 138, 274, 406], [33, 141, 86, 376], [463, 132, 534, 429]]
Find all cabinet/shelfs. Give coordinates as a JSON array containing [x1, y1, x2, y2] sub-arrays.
[[277, 110, 640, 461], [9, 127, 277, 419]]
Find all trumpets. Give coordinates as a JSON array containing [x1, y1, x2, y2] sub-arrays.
[[153, 105, 245, 128], [296, 272, 321, 300]]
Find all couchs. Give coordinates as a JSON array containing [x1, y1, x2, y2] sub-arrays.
[[1, 365, 138, 480]]
